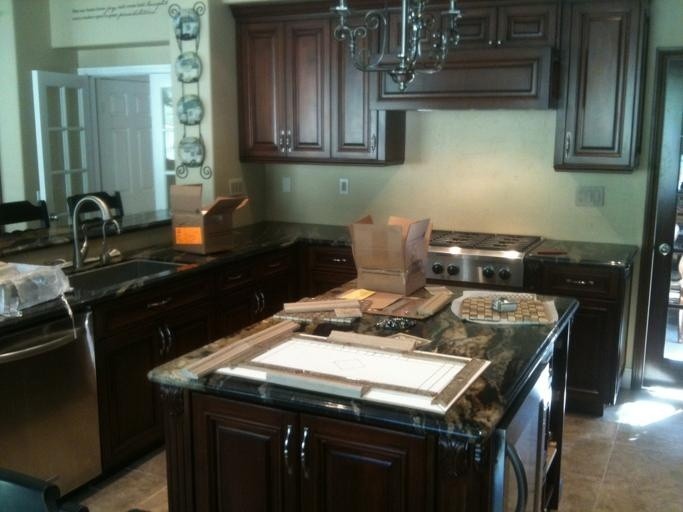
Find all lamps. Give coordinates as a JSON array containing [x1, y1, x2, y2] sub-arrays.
[[329, 0, 466, 91]]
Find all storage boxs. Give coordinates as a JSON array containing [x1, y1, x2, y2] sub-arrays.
[[170, 184, 250, 255], [348, 215, 433, 296]]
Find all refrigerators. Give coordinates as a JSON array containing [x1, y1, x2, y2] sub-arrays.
[[494, 363, 546, 511]]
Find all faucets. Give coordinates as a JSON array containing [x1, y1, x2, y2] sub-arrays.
[[100, 219, 121, 263], [72, 195, 110, 268]]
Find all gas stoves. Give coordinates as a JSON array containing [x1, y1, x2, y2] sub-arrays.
[[424, 229, 542, 287]]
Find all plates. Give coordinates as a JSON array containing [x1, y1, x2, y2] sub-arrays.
[[173, 8, 205, 167]]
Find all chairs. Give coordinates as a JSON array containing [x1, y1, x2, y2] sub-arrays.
[[65, 191, 124, 241], [1, 200, 50, 250]]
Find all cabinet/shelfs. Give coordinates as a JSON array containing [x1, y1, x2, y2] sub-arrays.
[[523, 262, 633, 416], [553, 1, 650, 174], [160, 386, 481, 511], [217, 244, 297, 340], [229, 0, 331, 164], [93, 268, 217, 480], [482, 322, 570, 511], [331, 0, 406, 167], [368, 1, 562, 110], [297, 243, 357, 301]]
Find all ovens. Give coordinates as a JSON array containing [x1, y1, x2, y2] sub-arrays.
[[523, 239, 637, 403]]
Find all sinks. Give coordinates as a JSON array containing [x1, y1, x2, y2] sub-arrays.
[[63, 256, 199, 290]]
